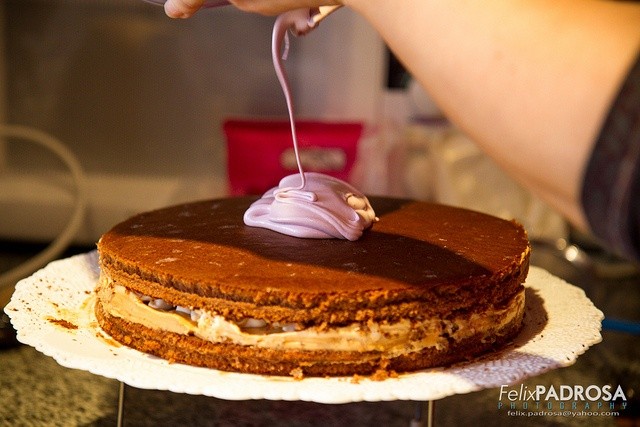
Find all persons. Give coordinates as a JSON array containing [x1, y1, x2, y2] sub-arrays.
[[164, 0, 640, 267]]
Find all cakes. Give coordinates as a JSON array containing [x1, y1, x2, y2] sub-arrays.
[[95, 10, 530, 375]]
